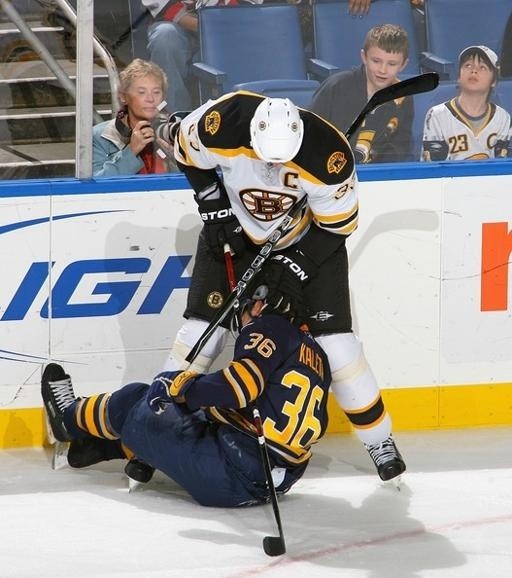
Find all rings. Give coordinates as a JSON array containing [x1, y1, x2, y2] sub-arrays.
[[143, 133, 147, 139]]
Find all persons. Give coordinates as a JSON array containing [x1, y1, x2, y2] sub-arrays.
[[125, 91, 407, 492], [310, 24, 414, 164], [93, 59, 181, 176], [420, 45, 512, 161], [142, 0, 272, 112], [43, 273, 333, 507]]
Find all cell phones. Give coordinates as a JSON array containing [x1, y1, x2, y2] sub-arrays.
[[146, 113, 182, 149]]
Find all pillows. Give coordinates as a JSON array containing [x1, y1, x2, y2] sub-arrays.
[[230, 296, 256, 339], [249, 97, 305, 164]]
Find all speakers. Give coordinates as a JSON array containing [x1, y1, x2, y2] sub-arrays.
[[41, 363, 77, 442], [68, 433, 127, 468]]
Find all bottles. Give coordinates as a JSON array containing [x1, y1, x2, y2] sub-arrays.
[[458, 46, 501, 69]]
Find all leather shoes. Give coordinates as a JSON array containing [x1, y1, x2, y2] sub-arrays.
[[147, 370, 205, 415], [194, 194, 245, 262], [248, 246, 318, 329]]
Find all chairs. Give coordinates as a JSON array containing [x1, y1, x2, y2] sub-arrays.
[[364, 433, 406, 481], [189, 0, 512, 157], [124, 454, 155, 483]]
